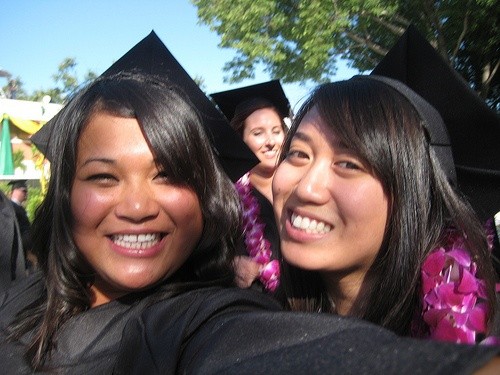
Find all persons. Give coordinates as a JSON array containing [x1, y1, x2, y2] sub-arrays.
[[230, 98, 324, 298], [271, 74, 500, 346], [6, 179, 31, 236], [0, 72, 500, 375]]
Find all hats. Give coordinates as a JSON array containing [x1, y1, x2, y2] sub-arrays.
[[7, 180, 27, 189], [352, 22, 500, 217], [30, 30, 261, 183], [209, 78, 293, 117]]
